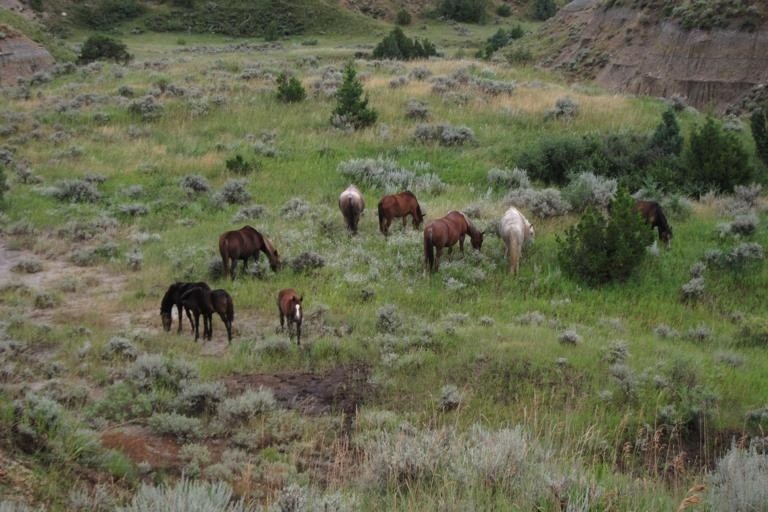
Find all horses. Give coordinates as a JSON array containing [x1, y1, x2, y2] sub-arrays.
[[422, 209, 486, 270], [497, 205, 536, 277], [180, 286, 234, 343], [377, 190, 427, 237], [218, 224, 282, 281], [337, 183, 365, 233], [606, 195, 673, 253], [159, 282, 209, 337], [277, 288, 303, 345]]
